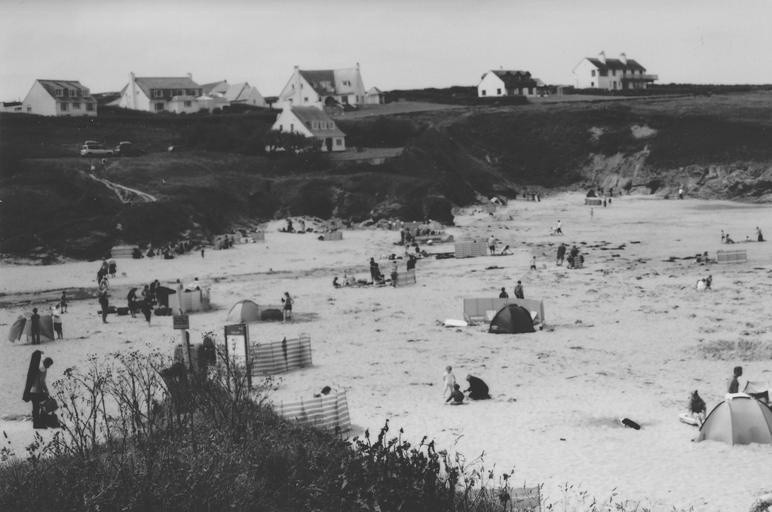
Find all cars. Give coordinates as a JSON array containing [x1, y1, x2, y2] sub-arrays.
[[168, 142, 195, 151]]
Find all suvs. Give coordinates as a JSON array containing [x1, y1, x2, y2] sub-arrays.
[[115, 140, 146, 157], [80, 140, 114, 158]]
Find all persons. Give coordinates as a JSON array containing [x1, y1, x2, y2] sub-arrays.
[[95, 234, 234, 324], [727, 366, 742, 393], [685, 389, 707, 429], [30, 307, 41, 344], [443, 366, 457, 397], [514, 280, 524, 299], [499, 287, 509, 298], [49, 303, 64, 338], [285, 214, 443, 288], [281, 292, 294, 323], [463, 374, 491, 399], [678, 187, 685, 199], [694, 224, 766, 291], [60, 290, 68, 314], [445, 384, 465, 404], [488, 183, 615, 270], [28, 355, 55, 430]]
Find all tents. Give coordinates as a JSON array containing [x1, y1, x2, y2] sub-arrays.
[[695, 393, 772, 446], [488, 303, 537, 333], [224, 298, 261, 323]]
